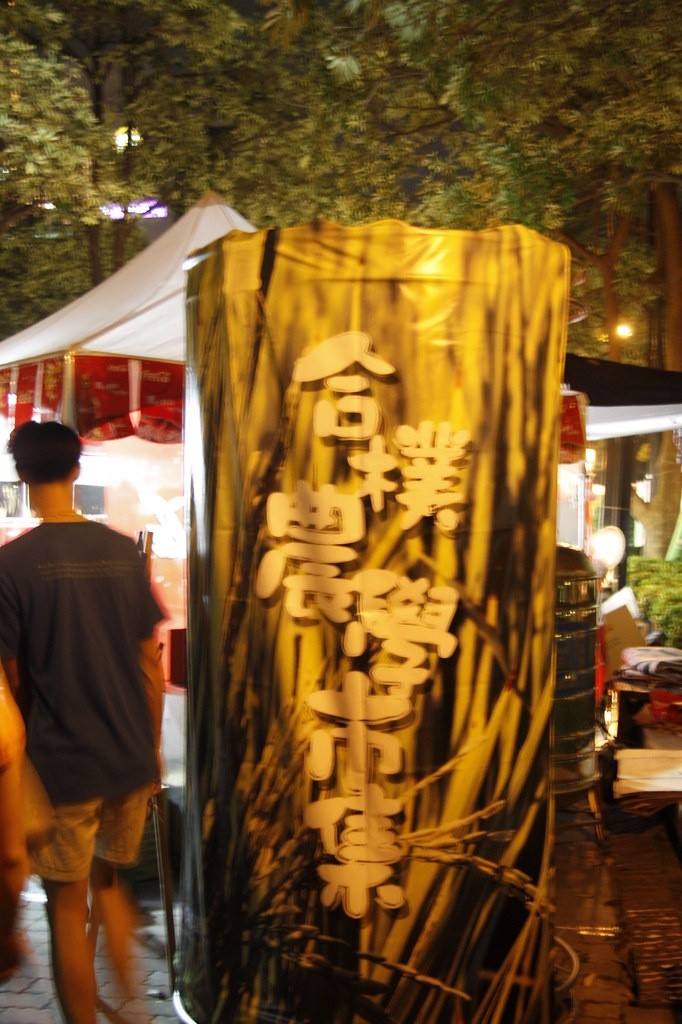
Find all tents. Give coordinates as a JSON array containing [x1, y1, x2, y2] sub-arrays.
[[0, 188, 682, 513]]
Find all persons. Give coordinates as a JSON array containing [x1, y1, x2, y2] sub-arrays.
[[1, 422, 170, 1024]]
